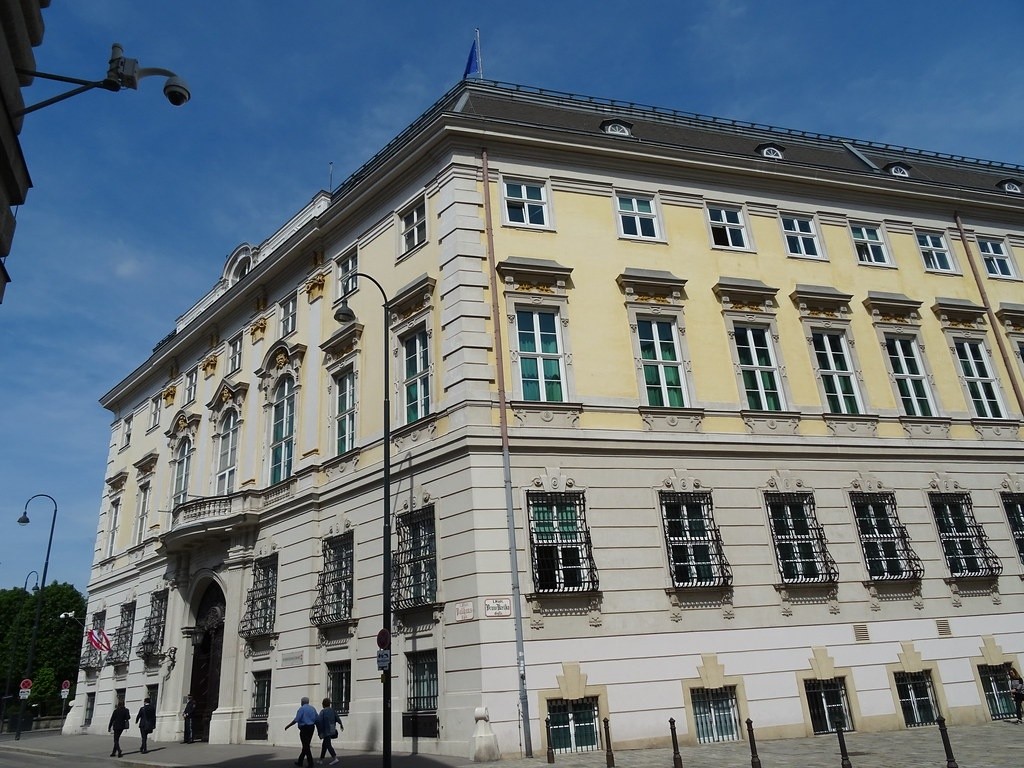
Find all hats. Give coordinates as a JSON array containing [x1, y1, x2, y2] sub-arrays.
[[188, 694, 193, 698]]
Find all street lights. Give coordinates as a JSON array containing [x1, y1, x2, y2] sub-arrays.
[[14, 494, 58, 740], [332, 274, 391, 768], [15, 571, 40, 663]]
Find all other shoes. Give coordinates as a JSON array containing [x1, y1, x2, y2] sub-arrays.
[[1016, 719, 1022, 724], [316, 760, 323, 765], [118, 749, 122, 758], [306, 762, 314, 768], [181, 740, 193, 744], [110, 754, 115, 756], [140, 748, 145, 754], [329, 758, 339, 765], [294, 761, 303, 767]]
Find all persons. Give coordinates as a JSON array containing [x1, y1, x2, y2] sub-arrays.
[[135, 698, 156, 754], [317, 698, 344, 765], [1009, 667, 1024, 724], [285, 697, 320, 767], [181, 694, 197, 744], [108, 701, 131, 758]]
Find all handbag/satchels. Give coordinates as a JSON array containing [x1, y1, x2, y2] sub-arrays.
[[330, 730, 338, 739], [123, 719, 129, 729], [144, 721, 153, 733]]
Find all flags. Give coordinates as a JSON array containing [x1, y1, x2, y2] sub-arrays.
[[463, 42, 478, 79], [87, 628, 111, 651]]
